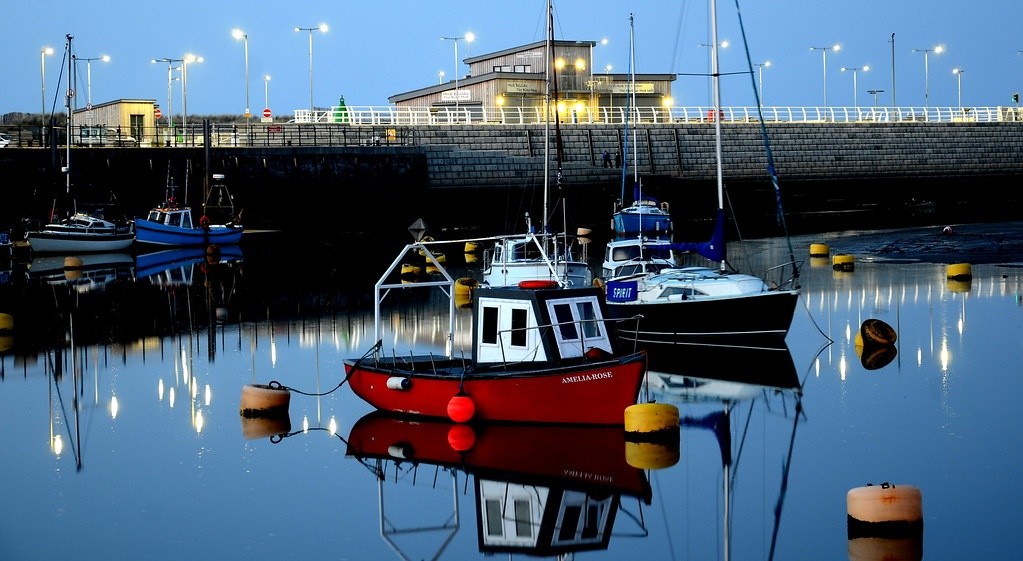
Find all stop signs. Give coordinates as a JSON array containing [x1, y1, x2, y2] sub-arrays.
[[263, 109, 271, 118], [154, 110, 162, 119]]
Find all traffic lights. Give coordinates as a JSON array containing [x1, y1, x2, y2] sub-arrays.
[[1013, 94, 1018, 103]]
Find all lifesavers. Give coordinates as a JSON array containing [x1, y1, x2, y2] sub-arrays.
[[518, 280, 558, 289]]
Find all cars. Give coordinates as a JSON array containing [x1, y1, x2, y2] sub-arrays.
[[76, 126, 138, 147], [0, 132, 9, 148]]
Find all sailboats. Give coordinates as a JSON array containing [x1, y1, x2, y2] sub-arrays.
[[22, 32, 138, 255], [337, 0, 688, 435], [599, 11, 805, 342]]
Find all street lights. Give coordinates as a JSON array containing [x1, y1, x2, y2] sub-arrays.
[[40, 47, 53, 148], [842, 66, 870, 120], [440, 34, 474, 124], [150, 57, 205, 140], [754, 62, 770, 105], [952, 68, 965, 115], [604, 64, 612, 75], [73, 56, 110, 148], [697, 42, 728, 105], [231, 30, 251, 147], [810, 45, 840, 121], [439, 71, 444, 86], [265, 75, 270, 114], [294, 23, 327, 122], [911, 46, 944, 116]]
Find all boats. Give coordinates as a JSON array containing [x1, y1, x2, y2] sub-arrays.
[[609, 338, 808, 561], [28, 249, 135, 308], [130, 159, 244, 245], [134, 245, 251, 328], [338, 409, 682, 561]]
